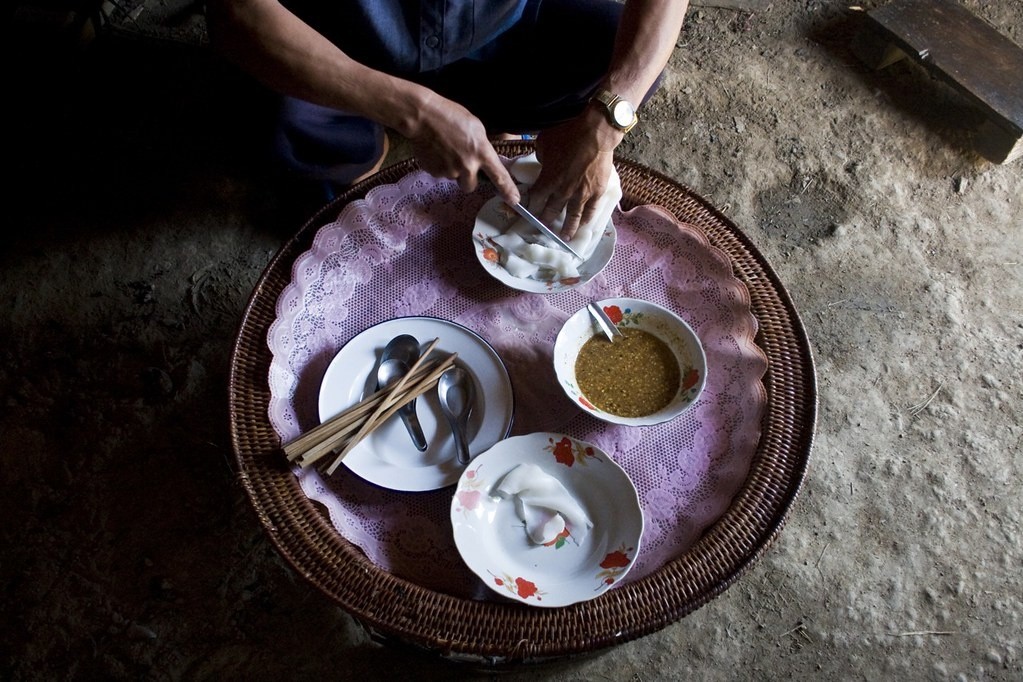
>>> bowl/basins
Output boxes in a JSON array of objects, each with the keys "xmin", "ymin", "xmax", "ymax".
[{"xmin": 553, "ymin": 296, "xmax": 707, "ymax": 425}]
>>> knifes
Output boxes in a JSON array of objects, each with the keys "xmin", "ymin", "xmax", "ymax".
[{"xmin": 478, "ymin": 170, "xmax": 580, "ymax": 260}]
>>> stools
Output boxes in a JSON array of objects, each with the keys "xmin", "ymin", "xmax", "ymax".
[{"xmin": 860, "ymin": 0, "xmax": 1023, "ymax": 168}]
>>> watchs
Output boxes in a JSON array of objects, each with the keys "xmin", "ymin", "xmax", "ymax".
[{"xmin": 589, "ymin": 88, "xmax": 638, "ymax": 133}]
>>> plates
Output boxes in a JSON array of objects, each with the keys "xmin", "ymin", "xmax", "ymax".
[
  {"xmin": 471, "ymin": 184, "xmax": 616, "ymax": 294},
  {"xmin": 449, "ymin": 432, "xmax": 644, "ymax": 608},
  {"xmin": 317, "ymin": 316, "xmax": 516, "ymax": 492}
]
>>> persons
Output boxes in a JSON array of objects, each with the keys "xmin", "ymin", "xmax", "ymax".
[{"xmin": 207, "ymin": 0, "xmax": 690, "ymax": 242}]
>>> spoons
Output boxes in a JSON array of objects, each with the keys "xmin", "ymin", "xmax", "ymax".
[
  {"xmin": 438, "ymin": 368, "xmax": 474, "ymax": 465},
  {"xmin": 374, "ymin": 333, "xmax": 421, "ymax": 394},
  {"xmin": 377, "ymin": 358, "xmax": 428, "ymax": 452}
]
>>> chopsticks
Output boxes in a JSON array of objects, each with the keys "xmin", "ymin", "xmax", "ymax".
[{"xmin": 279, "ymin": 336, "xmax": 458, "ymax": 477}]
